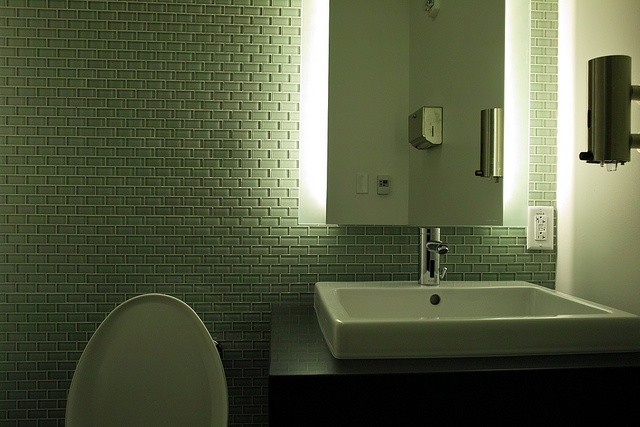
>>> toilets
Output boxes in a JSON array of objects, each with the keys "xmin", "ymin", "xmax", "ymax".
[{"xmin": 63, "ymin": 293, "xmax": 229, "ymax": 426}]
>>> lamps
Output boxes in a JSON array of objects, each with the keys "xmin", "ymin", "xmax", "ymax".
[
  {"xmin": 579, "ymin": 55, "xmax": 640, "ymax": 170},
  {"xmin": 475, "ymin": 108, "xmax": 503, "ymax": 182}
]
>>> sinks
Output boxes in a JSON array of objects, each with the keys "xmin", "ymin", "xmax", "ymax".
[{"xmin": 313, "ymin": 280, "xmax": 640, "ymax": 360}]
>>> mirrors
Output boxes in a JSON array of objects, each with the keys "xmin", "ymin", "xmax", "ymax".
[{"xmin": 298, "ymin": 0, "xmax": 532, "ymax": 226}]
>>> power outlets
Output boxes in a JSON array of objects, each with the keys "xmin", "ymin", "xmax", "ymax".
[{"xmin": 527, "ymin": 207, "xmax": 553, "ymax": 251}]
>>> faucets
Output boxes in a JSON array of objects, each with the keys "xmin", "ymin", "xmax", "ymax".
[{"xmin": 418, "ymin": 227, "xmax": 449, "ymax": 286}]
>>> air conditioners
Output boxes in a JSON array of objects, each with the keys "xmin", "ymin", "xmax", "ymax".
[{"xmin": 408, "ymin": 106, "xmax": 442, "ymax": 151}]
